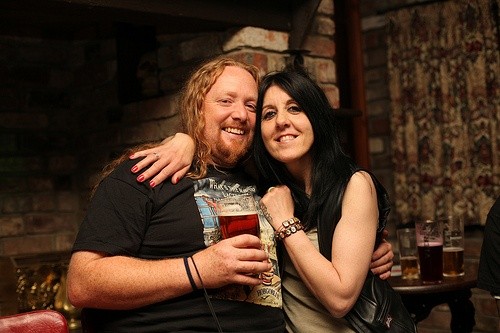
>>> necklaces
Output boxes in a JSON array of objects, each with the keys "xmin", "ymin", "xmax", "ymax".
[{"xmin": 306, "ymin": 192, "xmax": 312, "ymax": 198}]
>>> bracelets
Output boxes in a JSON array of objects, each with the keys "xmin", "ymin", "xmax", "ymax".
[
  {"xmin": 275, "ymin": 217, "xmax": 304, "ymax": 240},
  {"xmin": 184, "ymin": 255, "xmax": 222, "ymax": 333}
]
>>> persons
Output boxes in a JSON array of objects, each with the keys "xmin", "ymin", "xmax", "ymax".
[
  {"xmin": 129, "ymin": 65, "xmax": 418, "ymax": 333},
  {"xmin": 477, "ymin": 197, "xmax": 500, "ymax": 314},
  {"xmin": 66, "ymin": 58, "xmax": 395, "ymax": 333}
]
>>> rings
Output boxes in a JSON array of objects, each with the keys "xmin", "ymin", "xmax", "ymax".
[
  {"xmin": 267, "ymin": 187, "xmax": 275, "ymax": 192},
  {"xmin": 154, "ymin": 153, "xmax": 159, "ymax": 158}
]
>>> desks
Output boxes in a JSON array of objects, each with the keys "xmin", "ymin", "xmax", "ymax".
[{"xmin": 386, "ymin": 267, "xmax": 478, "ymax": 333}]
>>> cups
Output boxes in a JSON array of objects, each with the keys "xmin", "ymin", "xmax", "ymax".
[
  {"xmin": 398, "ymin": 229, "xmax": 419, "ymax": 287},
  {"xmin": 216, "ymin": 194, "xmax": 260, "ymax": 277},
  {"xmin": 415, "ymin": 219, "xmax": 445, "ymax": 285},
  {"xmin": 444, "ymin": 227, "xmax": 465, "ymax": 278},
  {"xmin": 16, "ymin": 265, "xmax": 81, "ymax": 331}
]
